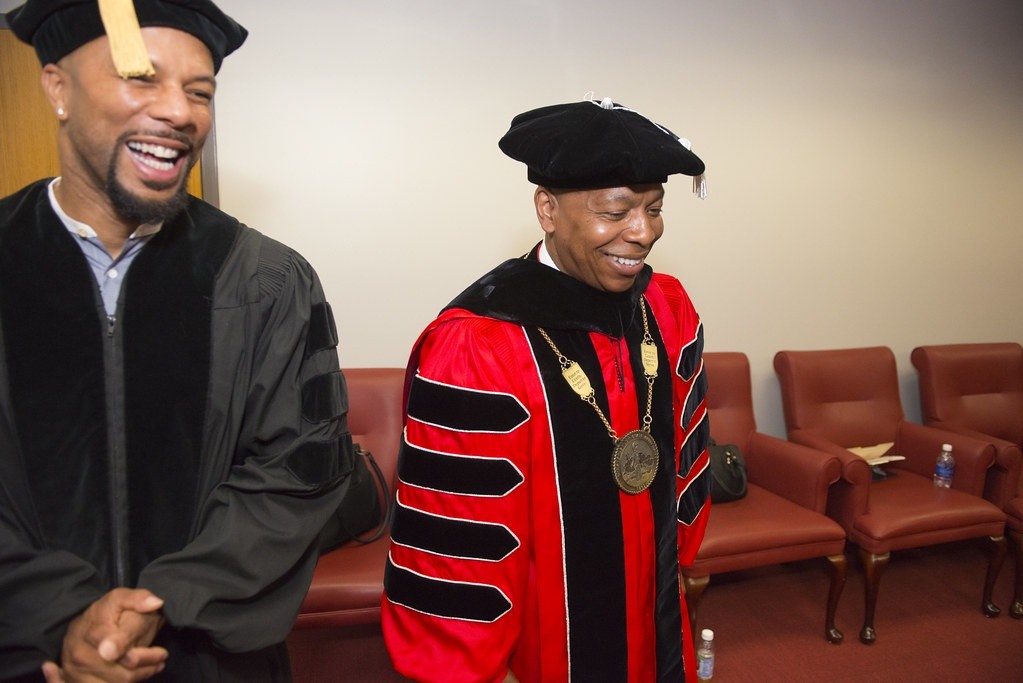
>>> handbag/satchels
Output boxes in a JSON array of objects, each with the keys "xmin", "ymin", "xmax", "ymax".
[
  {"xmin": 316, "ymin": 440, "xmax": 393, "ymax": 554},
  {"xmin": 704, "ymin": 438, "xmax": 747, "ymax": 501}
]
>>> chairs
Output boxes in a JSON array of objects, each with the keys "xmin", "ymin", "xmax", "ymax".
[
  {"xmin": 911, "ymin": 341, "xmax": 1023, "ymax": 619},
  {"xmin": 292, "ymin": 368, "xmax": 407, "ymax": 629},
  {"xmin": 775, "ymin": 344, "xmax": 1006, "ymax": 644},
  {"xmin": 683, "ymin": 352, "xmax": 848, "ymax": 643}
]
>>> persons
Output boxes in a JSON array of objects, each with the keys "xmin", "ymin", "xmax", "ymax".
[
  {"xmin": 0, "ymin": 0, "xmax": 360, "ymax": 683},
  {"xmin": 379, "ymin": 99, "xmax": 712, "ymax": 683}
]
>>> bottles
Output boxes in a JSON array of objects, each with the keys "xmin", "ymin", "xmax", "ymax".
[
  {"xmin": 933, "ymin": 443, "xmax": 955, "ymax": 489},
  {"xmin": 696, "ymin": 628, "xmax": 715, "ymax": 683}
]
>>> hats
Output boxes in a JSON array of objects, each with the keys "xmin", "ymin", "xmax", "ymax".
[
  {"xmin": 5, "ymin": 0, "xmax": 249, "ymax": 79},
  {"xmin": 498, "ymin": 96, "xmax": 708, "ymax": 201}
]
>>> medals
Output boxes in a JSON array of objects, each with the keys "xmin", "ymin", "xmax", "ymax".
[{"xmin": 613, "ymin": 428, "xmax": 660, "ymax": 494}]
